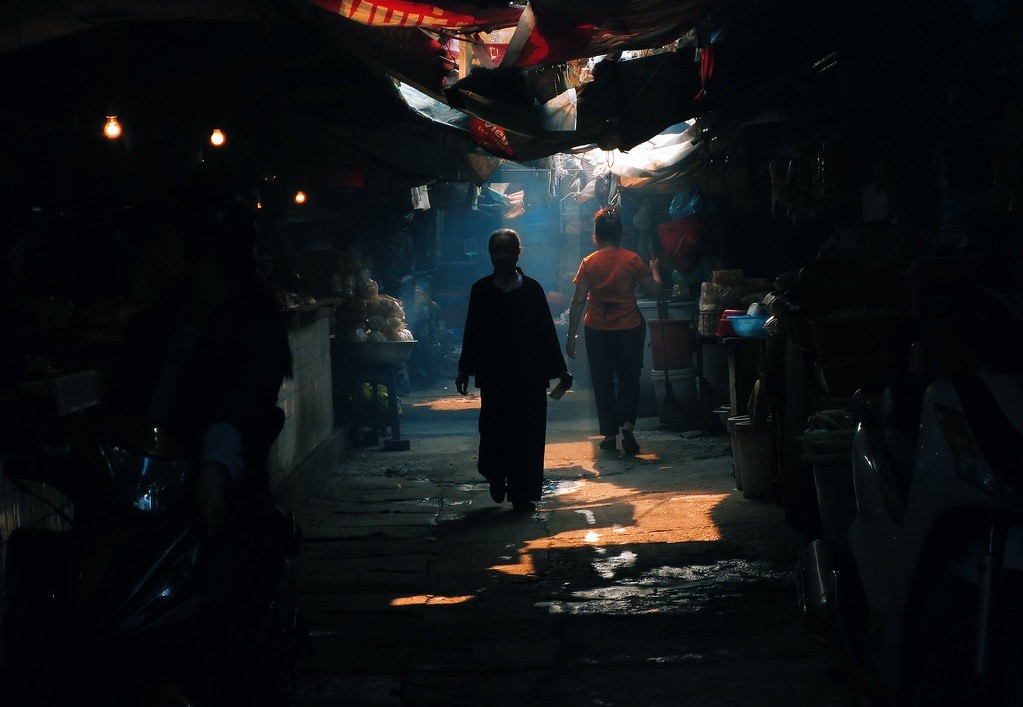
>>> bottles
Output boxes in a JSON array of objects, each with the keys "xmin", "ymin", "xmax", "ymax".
[{"xmin": 549, "ymin": 375, "xmax": 573, "ymax": 401}]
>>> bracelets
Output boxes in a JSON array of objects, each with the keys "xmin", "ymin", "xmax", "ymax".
[{"xmin": 567, "ymin": 333, "xmax": 578, "ymax": 339}]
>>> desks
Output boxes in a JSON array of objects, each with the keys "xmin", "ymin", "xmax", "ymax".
[{"xmin": 16, "ymin": 369, "xmax": 108, "ymax": 419}]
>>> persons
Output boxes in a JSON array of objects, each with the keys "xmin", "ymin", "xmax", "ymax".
[
  {"xmin": 456, "ymin": 228, "xmax": 573, "ymax": 513},
  {"xmin": 565, "ymin": 205, "xmax": 661, "ymax": 453}
]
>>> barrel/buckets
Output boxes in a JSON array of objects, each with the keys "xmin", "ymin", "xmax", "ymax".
[
  {"xmin": 647, "ymin": 318, "xmax": 692, "ymax": 370},
  {"xmin": 728, "ymin": 414, "xmax": 777, "ymax": 500},
  {"xmin": 651, "ymin": 370, "xmax": 700, "ymax": 430}
]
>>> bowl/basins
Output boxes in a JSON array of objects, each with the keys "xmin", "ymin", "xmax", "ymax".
[
  {"xmin": 332, "ymin": 340, "xmax": 419, "ymax": 367},
  {"xmin": 712, "ymin": 402, "xmax": 747, "ymax": 430},
  {"xmin": 726, "ymin": 314, "xmax": 770, "ymax": 337}
]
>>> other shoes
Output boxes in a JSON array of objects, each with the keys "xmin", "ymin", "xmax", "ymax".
[
  {"xmin": 598, "ymin": 438, "xmax": 639, "ymax": 454},
  {"xmin": 490, "ymin": 479, "xmax": 537, "ymax": 512}
]
